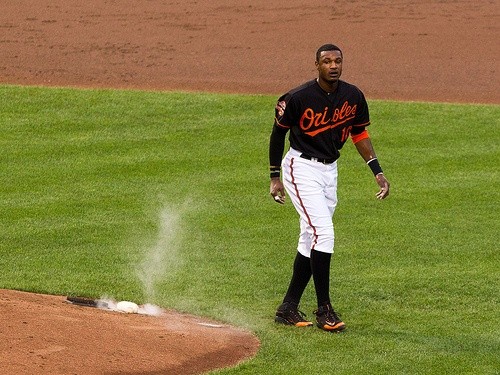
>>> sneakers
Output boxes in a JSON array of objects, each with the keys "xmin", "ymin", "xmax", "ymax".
[
  {"xmin": 313, "ymin": 302, "xmax": 346, "ymax": 333},
  {"xmin": 275, "ymin": 301, "xmax": 313, "ymax": 328}
]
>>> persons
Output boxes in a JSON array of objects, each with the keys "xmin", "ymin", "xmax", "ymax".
[{"xmin": 268, "ymin": 44, "xmax": 391, "ymax": 332}]
